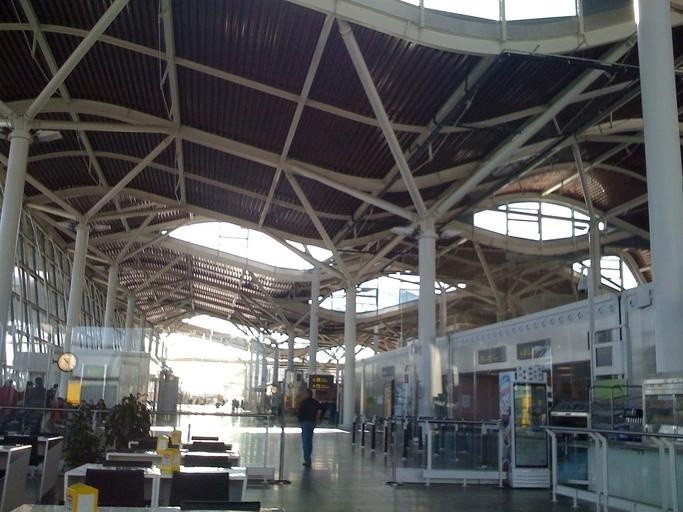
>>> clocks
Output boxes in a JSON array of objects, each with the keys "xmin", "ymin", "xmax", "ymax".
[{"xmin": 56, "ymin": 352, "xmax": 78, "ymax": 371}]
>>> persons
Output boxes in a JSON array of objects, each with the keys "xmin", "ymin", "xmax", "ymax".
[
  {"xmin": 86, "ymin": 399, "xmax": 100, "ymax": 410},
  {"xmin": 57, "ymin": 397, "xmax": 68, "ymax": 408},
  {"xmin": 29, "ymin": 377, "xmax": 46, "ymax": 415},
  {"xmin": 22, "ymin": 381, "xmax": 35, "ymax": 404},
  {"xmin": 296, "ymin": 387, "xmax": 327, "ymax": 468},
  {"xmin": 46, "ymin": 383, "xmax": 58, "ymax": 408},
  {"xmin": 96, "ymin": 398, "xmax": 107, "ymax": 409},
  {"xmin": 0, "ymin": 379, "xmax": 24, "ymax": 418},
  {"xmin": 78, "ymin": 399, "xmax": 91, "ymax": 409}
]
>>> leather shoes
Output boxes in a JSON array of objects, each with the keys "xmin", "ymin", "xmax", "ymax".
[{"xmin": 302, "ymin": 461, "xmax": 310, "ymax": 467}]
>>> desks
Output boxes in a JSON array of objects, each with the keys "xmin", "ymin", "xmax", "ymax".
[
  {"xmin": 63, "ymin": 440, "xmax": 248, "ymax": 507},
  {"xmin": 0, "ymin": 431, "xmax": 65, "ymax": 512}
]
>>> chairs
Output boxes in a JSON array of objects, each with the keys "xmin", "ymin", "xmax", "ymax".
[{"xmin": 85, "ymin": 437, "xmax": 230, "ymax": 507}]
